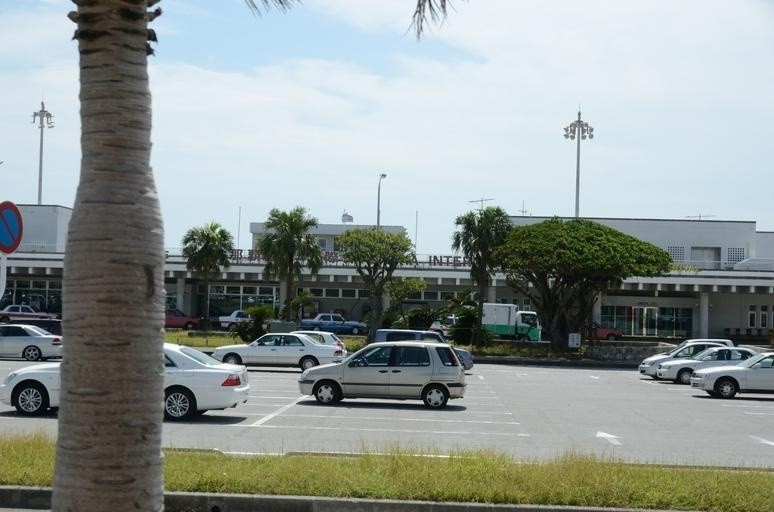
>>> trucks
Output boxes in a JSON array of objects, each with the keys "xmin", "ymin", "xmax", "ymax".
[{"xmin": 475, "ymin": 302, "xmax": 542, "ymax": 342}]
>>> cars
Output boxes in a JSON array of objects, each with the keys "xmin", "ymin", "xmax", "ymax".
[
  {"xmin": 637, "ymin": 338, "xmax": 774, "ymax": 399},
  {"xmin": 288, "ymin": 330, "xmax": 349, "ymax": 359},
  {"xmin": 211, "ymin": 333, "xmax": 345, "ymax": 372},
  {"xmin": 591, "ymin": 320, "xmax": 622, "ymax": 341},
  {"xmin": 300, "ymin": 313, "xmax": 368, "ymax": 336},
  {"xmin": 165, "ymin": 308, "xmax": 204, "ymax": 331},
  {"xmin": 218, "ymin": 310, "xmax": 268, "ymax": 330},
  {"xmin": 429, "ymin": 322, "xmax": 452, "ymax": 337},
  {"xmin": 298, "ymin": 341, "xmax": 466, "ymax": 410},
  {"xmin": 0, "ymin": 304, "xmax": 64, "ymax": 361},
  {"xmin": 23, "ymin": 293, "xmax": 46, "ymax": 304},
  {"xmin": 0, "ymin": 343, "xmax": 249, "ymax": 420}
]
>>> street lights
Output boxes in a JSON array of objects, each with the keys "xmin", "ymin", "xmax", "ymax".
[
  {"xmin": 564, "ymin": 109, "xmax": 595, "ymax": 218},
  {"xmin": 376, "ymin": 174, "xmax": 388, "ymax": 229},
  {"xmin": 32, "ymin": 98, "xmax": 55, "ymax": 206}
]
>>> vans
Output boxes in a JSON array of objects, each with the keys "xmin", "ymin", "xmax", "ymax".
[{"xmin": 375, "ymin": 328, "xmax": 473, "ymax": 372}]
